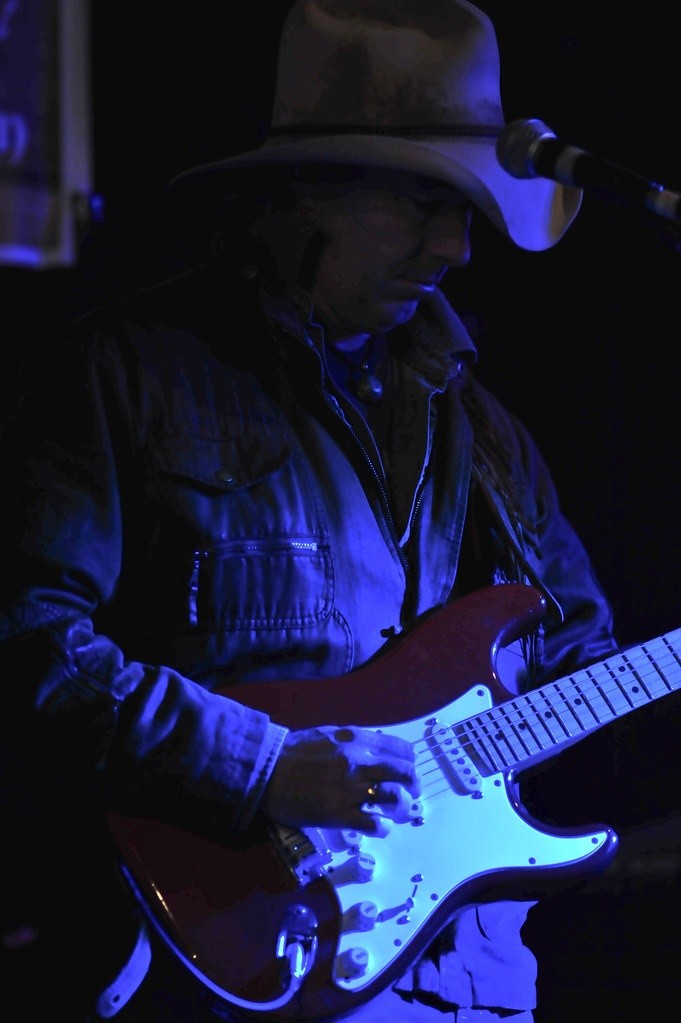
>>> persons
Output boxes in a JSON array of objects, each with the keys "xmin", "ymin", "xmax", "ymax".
[{"xmin": 0, "ymin": 0, "xmax": 627, "ymax": 1022}]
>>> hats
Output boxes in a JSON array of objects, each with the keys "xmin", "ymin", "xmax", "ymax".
[{"xmin": 169, "ymin": 0, "xmax": 587, "ymax": 252}]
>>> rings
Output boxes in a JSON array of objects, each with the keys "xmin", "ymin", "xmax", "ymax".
[{"xmin": 367, "ymin": 780, "xmax": 381, "ymax": 807}]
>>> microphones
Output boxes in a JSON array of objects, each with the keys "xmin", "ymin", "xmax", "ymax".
[{"xmin": 496, "ymin": 119, "xmax": 681, "ymax": 224}]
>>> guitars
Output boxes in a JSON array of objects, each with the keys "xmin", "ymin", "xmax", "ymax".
[{"xmin": 100, "ymin": 570, "xmax": 681, "ymax": 1022}]
